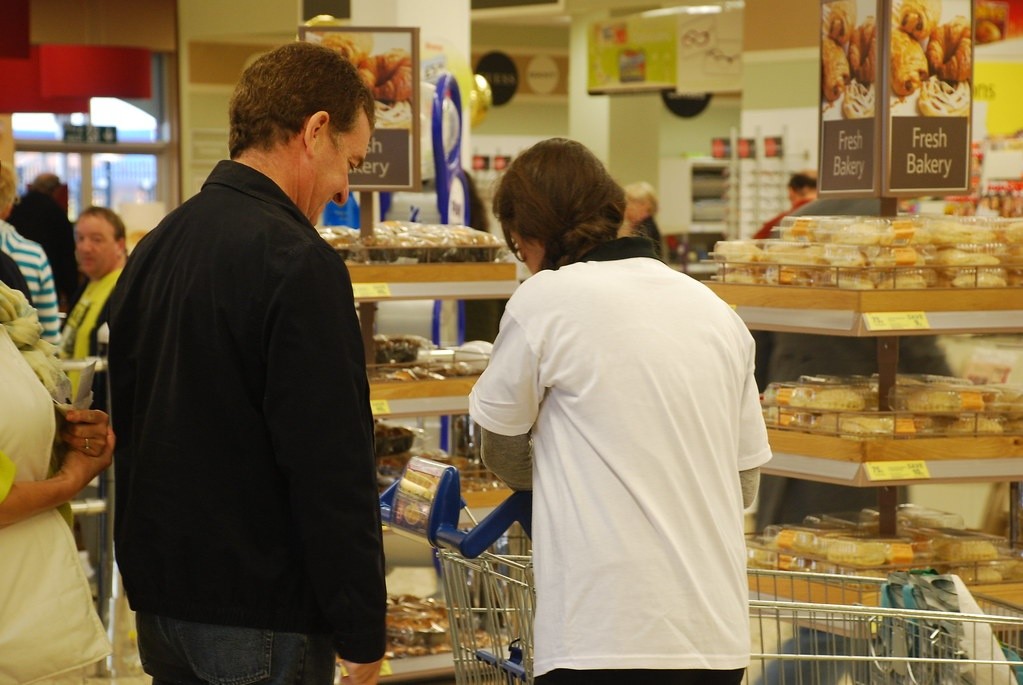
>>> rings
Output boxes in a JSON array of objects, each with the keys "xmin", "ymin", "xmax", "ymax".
[{"xmin": 85, "ymin": 438, "xmax": 89, "ymax": 449}]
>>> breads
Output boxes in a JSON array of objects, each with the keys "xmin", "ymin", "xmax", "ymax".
[
  {"xmin": 316, "ymin": 32, "xmax": 414, "ymax": 104},
  {"xmin": 758, "ymin": 364, "xmax": 1022, "ymax": 435},
  {"xmin": 745, "ymin": 511, "xmax": 1023, "ymax": 586},
  {"xmin": 710, "ymin": 215, "xmax": 1023, "ymax": 290},
  {"xmin": 822, "ymin": 0, "xmax": 973, "ymax": 119}
]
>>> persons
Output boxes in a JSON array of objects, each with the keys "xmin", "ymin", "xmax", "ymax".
[
  {"xmin": 0, "ymin": 280, "xmax": 116, "ymax": 685},
  {"xmin": 622, "ymin": 181, "xmax": 661, "ymax": 255},
  {"xmin": 110, "ymin": 41, "xmax": 388, "ymax": 685},
  {"xmin": 60, "ymin": 207, "xmax": 129, "ymax": 357},
  {"xmin": 753, "ymin": 172, "xmax": 817, "ymax": 238},
  {"xmin": 467, "ymin": 138, "xmax": 773, "ymax": 685},
  {"xmin": 6, "ymin": 174, "xmax": 80, "ymax": 300},
  {"xmin": 0, "ymin": 158, "xmax": 61, "ymax": 347}
]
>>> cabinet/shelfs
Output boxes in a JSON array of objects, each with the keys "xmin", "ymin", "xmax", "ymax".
[
  {"xmin": 704, "ymin": 255, "xmax": 1023, "ymax": 643},
  {"xmin": 336, "ymin": 262, "xmax": 521, "ymax": 685}
]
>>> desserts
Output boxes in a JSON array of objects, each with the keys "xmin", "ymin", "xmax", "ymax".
[
  {"xmin": 374, "ymin": 417, "xmax": 413, "ymax": 480},
  {"xmin": 380, "ymin": 590, "xmax": 506, "ymax": 658},
  {"xmin": 370, "ymin": 331, "xmax": 423, "ymax": 365},
  {"xmin": 316, "ymin": 220, "xmax": 507, "ymax": 265}
]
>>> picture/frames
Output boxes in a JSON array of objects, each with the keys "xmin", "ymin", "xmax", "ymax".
[
  {"xmin": 817, "ymin": 0, "xmax": 975, "ymax": 197},
  {"xmin": 298, "ymin": 26, "xmax": 422, "ymax": 191}
]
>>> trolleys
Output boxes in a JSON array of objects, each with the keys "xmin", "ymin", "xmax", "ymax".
[{"xmin": 378, "ymin": 457, "xmax": 1023, "ymax": 685}]
